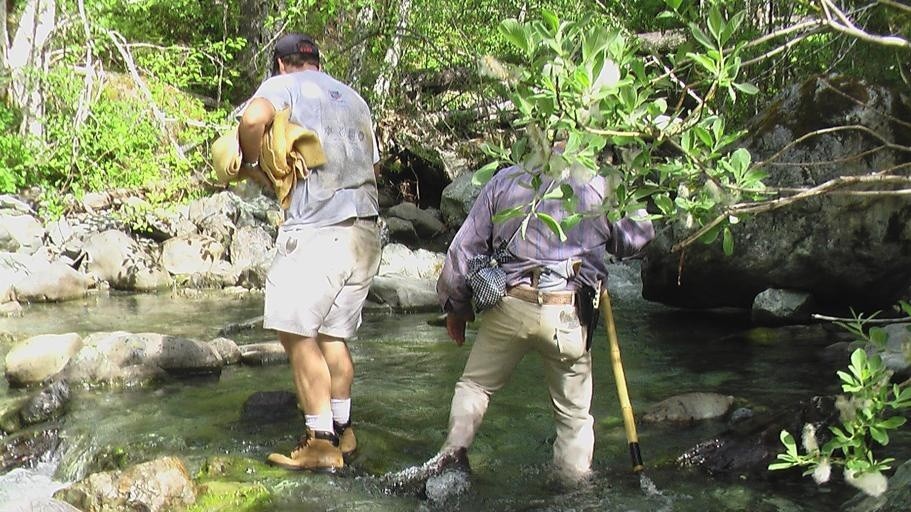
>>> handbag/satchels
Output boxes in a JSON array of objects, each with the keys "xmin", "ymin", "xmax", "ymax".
[{"xmin": 465, "ymin": 245, "xmax": 513, "ymax": 312}]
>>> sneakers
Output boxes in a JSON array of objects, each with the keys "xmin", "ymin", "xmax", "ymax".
[{"xmin": 267, "ymin": 414, "xmax": 356, "ymax": 475}]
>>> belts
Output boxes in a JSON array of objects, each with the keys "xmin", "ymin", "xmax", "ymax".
[{"xmin": 507, "ymin": 285, "xmax": 579, "ymax": 305}]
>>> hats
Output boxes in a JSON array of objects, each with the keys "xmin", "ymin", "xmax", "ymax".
[{"xmin": 272, "ymin": 33, "xmax": 320, "ymax": 75}]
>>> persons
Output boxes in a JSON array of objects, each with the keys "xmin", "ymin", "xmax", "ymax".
[
  {"xmin": 435, "ymin": 125, "xmax": 656, "ymax": 485},
  {"xmin": 237, "ymin": 32, "xmax": 386, "ymax": 471}
]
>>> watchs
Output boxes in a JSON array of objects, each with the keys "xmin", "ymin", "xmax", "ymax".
[{"xmin": 240, "ymin": 155, "xmax": 262, "ymax": 172}]
